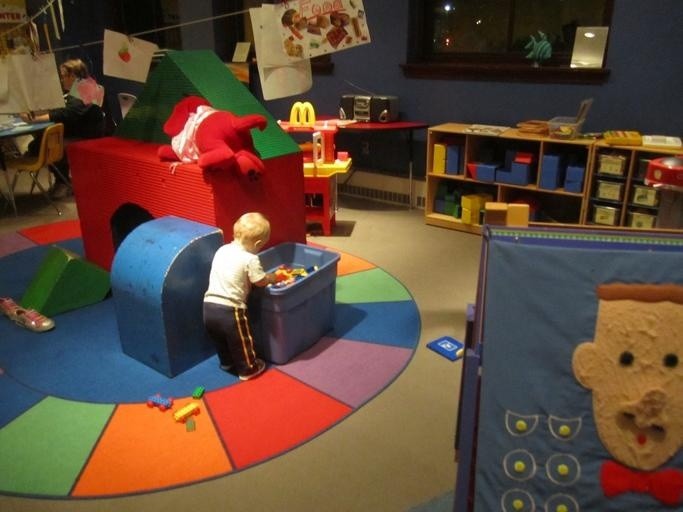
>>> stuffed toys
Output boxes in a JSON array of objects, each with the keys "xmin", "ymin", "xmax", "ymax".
[{"xmin": 156, "ymin": 95, "xmax": 272, "ymax": 188}]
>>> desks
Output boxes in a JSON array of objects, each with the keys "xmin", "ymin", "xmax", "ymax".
[
  {"xmin": 273, "ymin": 113, "xmax": 429, "ymax": 215},
  {"xmin": 0, "ymin": 118, "xmax": 58, "ymax": 199}
]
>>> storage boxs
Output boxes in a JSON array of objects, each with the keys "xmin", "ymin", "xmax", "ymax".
[{"xmin": 242, "ymin": 240, "xmax": 342, "ymax": 369}]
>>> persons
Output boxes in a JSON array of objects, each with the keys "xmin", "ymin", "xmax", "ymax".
[
  {"xmin": 201, "ymin": 212, "xmax": 278, "ymax": 382},
  {"xmin": 21, "ymin": 58, "xmax": 117, "ymax": 199}
]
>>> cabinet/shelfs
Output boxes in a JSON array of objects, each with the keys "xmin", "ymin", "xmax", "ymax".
[
  {"xmin": 422, "ymin": 121, "xmax": 597, "ymax": 242},
  {"xmin": 582, "ymin": 134, "xmax": 683, "ymax": 232}
]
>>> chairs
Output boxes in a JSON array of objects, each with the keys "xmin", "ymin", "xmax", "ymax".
[
  {"xmin": 0, "ymin": 141, "xmax": 23, "ymax": 221},
  {"xmin": 3, "ymin": 121, "xmax": 73, "ymax": 218},
  {"xmin": 116, "ymin": 90, "xmax": 136, "ymax": 120}
]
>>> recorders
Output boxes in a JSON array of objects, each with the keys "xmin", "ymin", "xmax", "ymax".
[{"xmin": 339, "ymin": 92, "xmax": 400, "ymax": 123}]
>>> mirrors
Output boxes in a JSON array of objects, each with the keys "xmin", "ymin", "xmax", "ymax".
[{"xmin": 568, "ymin": 25, "xmax": 610, "ymax": 71}]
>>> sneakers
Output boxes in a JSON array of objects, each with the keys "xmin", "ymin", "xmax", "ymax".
[
  {"xmin": 236, "ymin": 357, "xmax": 267, "ymax": 382},
  {"xmin": 218, "ymin": 363, "xmax": 233, "ymax": 371}
]
